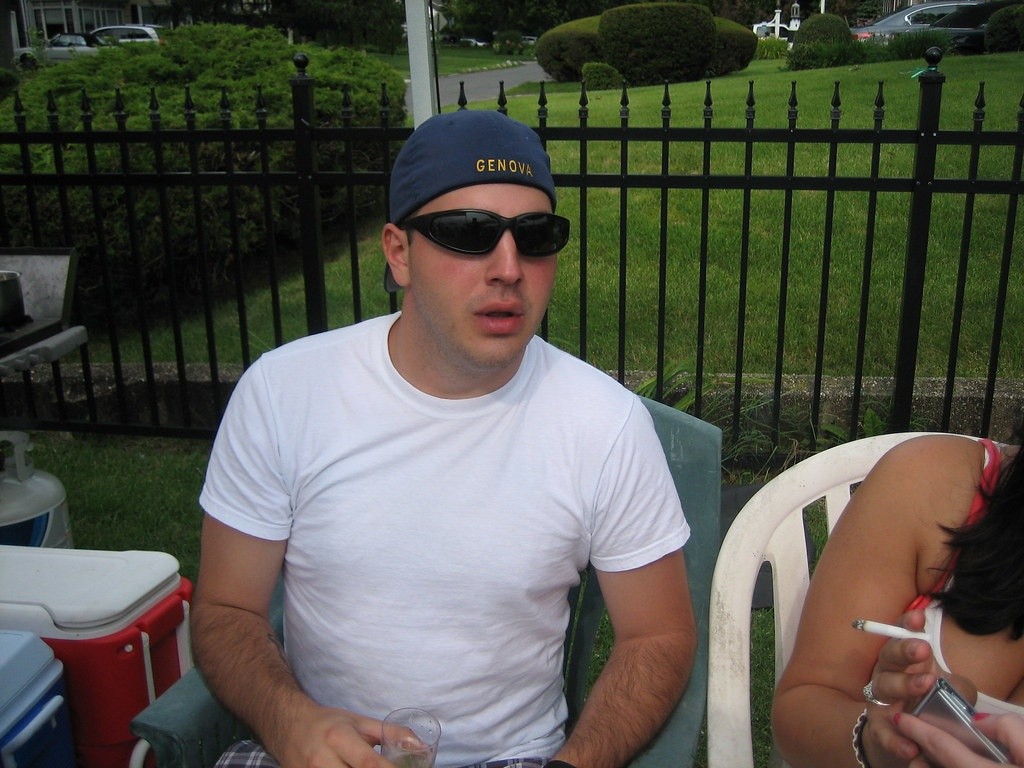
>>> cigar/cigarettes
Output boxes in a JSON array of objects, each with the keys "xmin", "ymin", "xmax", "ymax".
[{"xmin": 851, "ymin": 616, "xmax": 930, "ymax": 641}]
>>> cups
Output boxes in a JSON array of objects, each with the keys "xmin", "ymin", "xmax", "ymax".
[{"xmin": 380, "ymin": 707, "xmax": 442, "ymax": 768}]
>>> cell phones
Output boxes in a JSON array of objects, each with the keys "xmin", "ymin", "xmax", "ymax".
[{"xmin": 911, "ymin": 677, "xmax": 1014, "ymax": 764}]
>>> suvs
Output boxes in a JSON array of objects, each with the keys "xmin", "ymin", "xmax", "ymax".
[{"xmin": 89, "ymin": 23, "xmax": 165, "ymax": 46}]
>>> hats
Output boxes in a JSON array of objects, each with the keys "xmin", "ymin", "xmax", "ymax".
[{"xmin": 388, "ymin": 109, "xmax": 557, "ymax": 223}]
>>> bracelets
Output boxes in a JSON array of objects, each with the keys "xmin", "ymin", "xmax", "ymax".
[{"xmin": 852, "ymin": 707, "xmax": 869, "ymax": 768}]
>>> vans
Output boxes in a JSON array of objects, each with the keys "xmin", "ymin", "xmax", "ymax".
[{"xmin": 751, "ymin": 23, "xmax": 791, "ymax": 43}]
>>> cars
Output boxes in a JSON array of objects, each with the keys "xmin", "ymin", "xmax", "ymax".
[
  {"xmin": 850, "ymin": 0, "xmax": 989, "ymax": 56},
  {"xmin": 459, "ymin": 38, "xmax": 489, "ymax": 48},
  {"xmin": 12, "ymin": 32, "xmax": 118, "ymax": 72},
  {"xmin": 522, "ymin": 36, "xmax": 539, "ymax": 46}
]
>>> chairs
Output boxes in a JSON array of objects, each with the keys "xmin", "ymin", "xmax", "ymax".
[
  {"xmin": 128, "ymin": 395, "xmax": 720, "ymax": 768},
  {"xmin": 709, "ymin": 431, "xmax": 993, "ymax": 768}
]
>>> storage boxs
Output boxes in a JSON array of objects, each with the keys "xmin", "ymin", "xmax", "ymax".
[{"xmin": 0, "ymin": 542, "xmax": 194, "ymax": 768}]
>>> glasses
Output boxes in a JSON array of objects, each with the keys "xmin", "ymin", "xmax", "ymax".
[{"xmin": 398, "ymin": 208, "xmax": 571, "ymax": 257}]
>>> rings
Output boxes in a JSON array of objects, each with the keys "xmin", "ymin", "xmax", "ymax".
[{"xmin": 863, "ymin": 681, "xmax": 891, "ymax": 706}]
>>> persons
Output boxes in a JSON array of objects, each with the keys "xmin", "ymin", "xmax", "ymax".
[
  {"xmin": 771, "ymin": 396, "xmax": 1022, "ymax": 768},
  {"xmin": 191, "ymin": 114, "xmax": 697, "ymax": 768}
]
